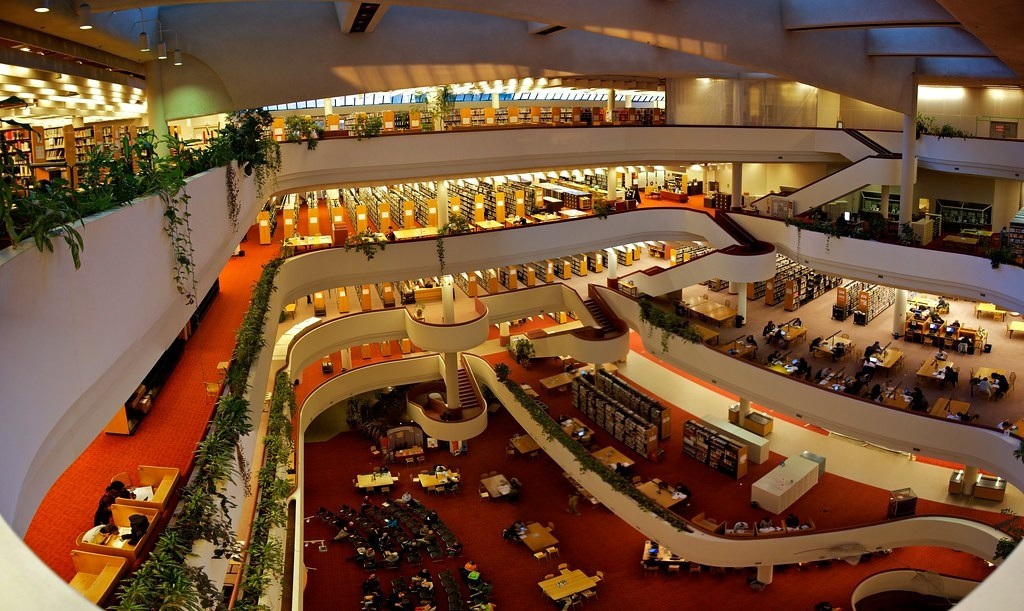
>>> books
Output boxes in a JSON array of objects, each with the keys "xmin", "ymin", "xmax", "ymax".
[
  {"xmin": 0, "ymin": 126, "xmax": 149, "ymax": 195},
  {"xmin": 314, "ymin": 244, "xmax": 637, "ymax": 318},
  {"xmin": 261, "ymin": 108, "xmax": 664, "ymax": 140},
  {"xmin": 683, "ymin": 422, "xmax": 738, "ymax": 478},
  {"xmin": 263, "ymin": 164, "xmax": 683, "ymax": 237},
  {"xmin": 571, "ymin": 372, "xmax": 663, "ymax": 460},
  {"xmin": 651, "ymin": 239, "xmax": 896, "ymax": 323}
]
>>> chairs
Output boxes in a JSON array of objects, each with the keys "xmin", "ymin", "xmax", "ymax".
[
  {"xmin": 111, "ymin": 472, "xmax": 133, "ymax": 488},
  {"xmin": 76, "ymin": 532, "xmax": 85, "ymax": 546},
  {"xmin": 317, "ymin": 446, "xmax": 680, "ymax": 611},
  {"xmin": 204, "ymin": 380, "xmax": 220, "ymax": 404}
]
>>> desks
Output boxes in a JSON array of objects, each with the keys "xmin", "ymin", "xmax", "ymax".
[
  {"xmin": 282, "ymin": 211, "xmax": 589, "ymax": 254},
  {"xmin": 511, "ymin": 418, "xmax": 686, "ymax": 600},
  {"xmin": 678, "ymin": 296, "xmax": 1024, "ymax": 440},
  {"xmin": 520, "ymin": 361, "xmax": 617, "ymax": 398},
  {"xmin": 396, "ymin": 447, "xmax": 423, "ymax": 458},
  {"xmin": 357, "ymin": 471, "xmax": 396, "ymax": 488},
  {"xmin": 943, "ymin": 229, "xmax": 993, "ymax": 251},
  {"xmin": 217, "ymin": 362, "xmax": 229, "ymax": 379},
  {"xmin": 481, "ymin": 474, "xmax": 513, "ymax": 498},
  {"xmin": 67, "ymin": 465, "xmax": 192, "ymax": 605},
  {"xmin": 418, "ymin": 470, "xmax": 458, "ymax": 487},
  {"xmin": 510, "ymin": 435, "xmax": 542, "ymax": 453}
]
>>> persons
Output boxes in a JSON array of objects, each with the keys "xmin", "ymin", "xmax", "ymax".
[
  {"xmin": 674, "ymin": 179, "xmax": 681, "ymax": 194},
  {"xmin": 759, "ymin": 516, "xmax": 773, "ymax": 528},
  {"xmin": 676, "ymin": 482, "xmax": 692, "ymax": 505},
  {"xmin": 365, "ymin": 226, "xmax": 375, "ymax": 236},
  {"xmin": 331, "ymin": 444, "xmax": 581, "ymax": 611},
  {"xmin": 82, "ymin": 524, "xmax": 120, "ymax": 546},
  {"xmin": 815, "ymin": 602, "xmax": 832, "ymax": 611},
  {"xmin": 385, "ymin": 226, "xmax": 395, "ymax": 237},
  {"xmin": 519, "ymin": 217, "xmax": 527, "ymax": 226},
  {"xmin": 96, "ymin": 480, "xmax": 136, "ymax": 525},
  {"xmin": 615, "ymin": 462, "xmax": 630, "ymax": 478},
  {"xmin": 734, "ymin": 521, "xmax": 749, "ymax": 530},
  {"xmin": 306, "ymin": 295, "xmax": 312, "ymax": 305},
  {"xmin": 745, "ymin": 297, "xmax": 1011, "ymax": 430},
  {"xmin": 627, "ymin": 185, "xmax": 636, "ymax": 199},
  {"xmin": 786, "ymin": 514, "xmax": 800, "ymax": 528},
  {"xmin": 292, "ymin": 229, "xmax": 300, "ymax": 237}
]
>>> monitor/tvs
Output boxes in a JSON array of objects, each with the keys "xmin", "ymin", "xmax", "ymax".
[
  {"xmin": 912, "ymin": 321, "xmax": 916, "ymax": 325},
  {"xmin": 930, "ymin": 324, "xmax": 938, "ymax": 330},
  {"xmin": 946, "ymin": 327, "xmax": 955, "ymax": 332}
]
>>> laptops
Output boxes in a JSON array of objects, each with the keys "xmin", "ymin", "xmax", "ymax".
[
  {"xmin": 791, "ymin": 358, "xmax": 799, "ymax": 366},
  {"xmin": 905, "ymin": 387, "xmax": 912, "ymax": 395}
]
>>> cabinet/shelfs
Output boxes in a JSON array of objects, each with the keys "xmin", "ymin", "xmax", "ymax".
[
  {"xmin": 572, "ymin": 369, "xmax": 749, "ymax": 479},
  {"xmin": 0, "ymin": 116, "xmax": 180, "ymax": 207},
  {"xmin": 747, "ymin": 251, "xmax": 894, "ymax": 324},
  {"xmin": 262, "ymin": 107, "xmax": 666, "ymax": 142},
  {"xmin": 258, "ymin": 180, "xmax": 544, "ymax": 244}
]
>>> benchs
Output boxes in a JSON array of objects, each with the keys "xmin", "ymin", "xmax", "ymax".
[{"xmin": 692, "ymin": 512, "xmax": 816, "ymax": 539}]
